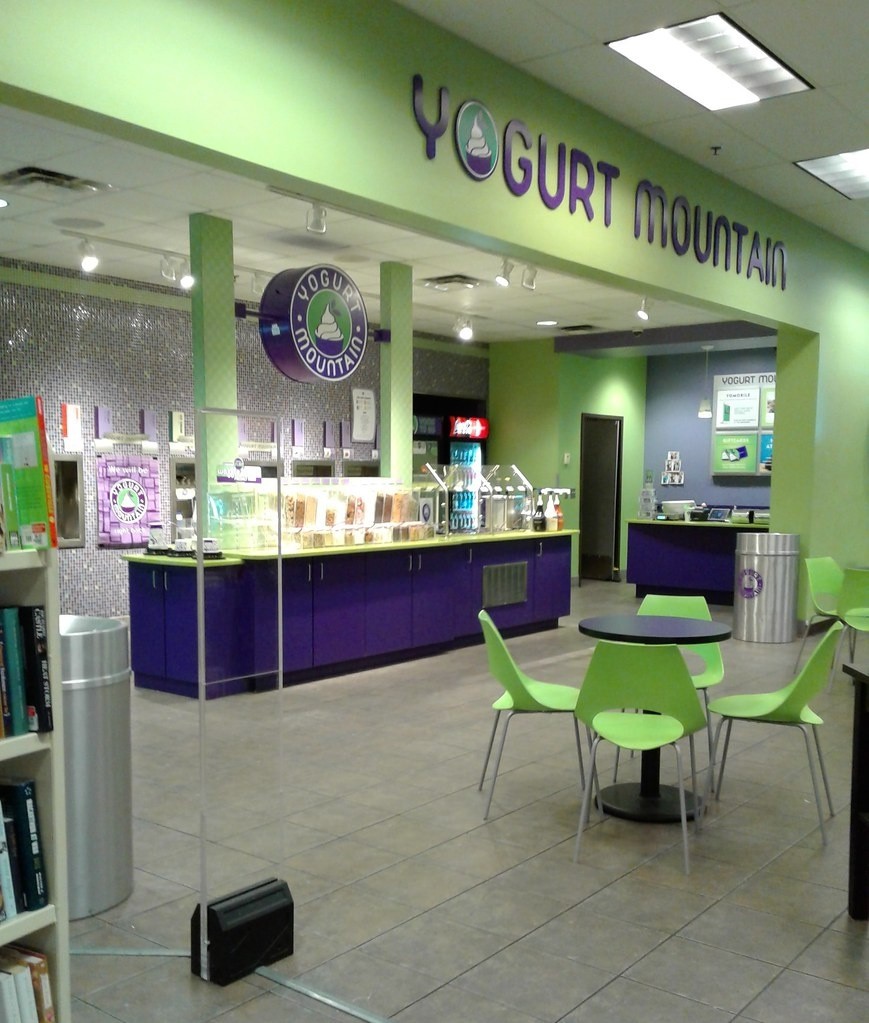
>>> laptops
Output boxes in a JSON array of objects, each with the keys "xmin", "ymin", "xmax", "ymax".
[{"xmin": 707, "ymin": 508, "xmax": 730, "ymax": 521}]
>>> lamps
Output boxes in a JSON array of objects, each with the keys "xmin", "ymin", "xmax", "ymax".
[
  {"xmin": 496, "ymin": 257, "xmax": 514, "ymax": 286},
  {"xmin": 180, "ymin": 257, "xmax": 193, "ymax": 288},
  {"xmin": 253, "ymin": 272, "xmax": 265, "ymax": 294},
  {"xmin": 637, "ymin": 297, "xmax": 650, "ymax": 320},
  {"xmin": 521, "ymin": 265, "xmax": 537, "ymax": 289},
  {"xmin": 452, "ymin": 314, "xmax": 473, "ymax": 340},
  {"xmin": 305, "ymin": 201, "xmax": 328, "ymax": 232},
  {"xmin": 77, "ymin": 238, "xmax": 98, "ymax": 270},
  {"xmin": 698, "ymin": 345, "xmax": 715, "ymax": 417}
]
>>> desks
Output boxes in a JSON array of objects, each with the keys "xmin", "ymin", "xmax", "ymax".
[
  {"xmin": 579, "ymin": 615, "xmax": 732, "ymax": 823},
  {"xmin": 624, "ymin": 519, "xmax": 769, "ymax": 606}
]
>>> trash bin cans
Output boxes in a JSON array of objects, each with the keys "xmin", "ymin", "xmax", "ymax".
[
  {"xmin": 58, "ymin": 612, "xmax": 134, "ymax": 923},
  {"xmin": 735, "ymin": 529, "xmax": 799, "ymax": 645}
]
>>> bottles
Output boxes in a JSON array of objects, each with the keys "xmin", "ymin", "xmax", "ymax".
[
  {"xmin": 176, "ymin": 511, "xmax": 184, "ymax": 528},
  {"xmin": 533, "ymin": 492, "xmax": 563, "ymax": 532},
  {"xmin": 149, "ymin": 525, "xmax": 164, "ymax": 549}
]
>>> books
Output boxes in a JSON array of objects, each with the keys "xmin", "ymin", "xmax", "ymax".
[
  {"xmin": 0, "ymin": 775, "xmax": 49, "ymax": 923},
  {"xmin": 0, "ymin": 942, "xmax": 56, "ymax": 1023},
  {"xmin": 0, "ymin": 605, "xmax": 54, "ymax": 738}
]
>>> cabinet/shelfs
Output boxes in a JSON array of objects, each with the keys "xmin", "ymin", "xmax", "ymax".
[
  {"xmin": 121, "ymin": 529, "xmax": 580, "ymax": 701},
  {"xmin": 0, "ymin": 420, "xmax": 72, "ymax": 1023}
]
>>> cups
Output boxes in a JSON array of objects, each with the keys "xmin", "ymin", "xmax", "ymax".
[
  {"xmin": 175, "ymin": 539, "xmax": 192, "ymax": 551},
  {"xmin": 203, "ymin": 539, "xmax": 219, "ymax": 553}
]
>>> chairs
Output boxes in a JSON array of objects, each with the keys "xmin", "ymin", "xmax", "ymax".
[
  {"xmin": 479, "ymin": 608, "xmax": 603, "ymax": 822},
  {"xmin": 793, "ymin": 556, "xmax": 869, "ymax": 693},
  {"xmin": 630, "ymin": 595, "xmax": 724, "ymax": 765},
  {"xmin": 707, "ymin": 621, "xmax": 843, "ymax": 845},
  {"xmin": 573, "ymin": 639, "xmax": 709, "ymax": 875}
]
